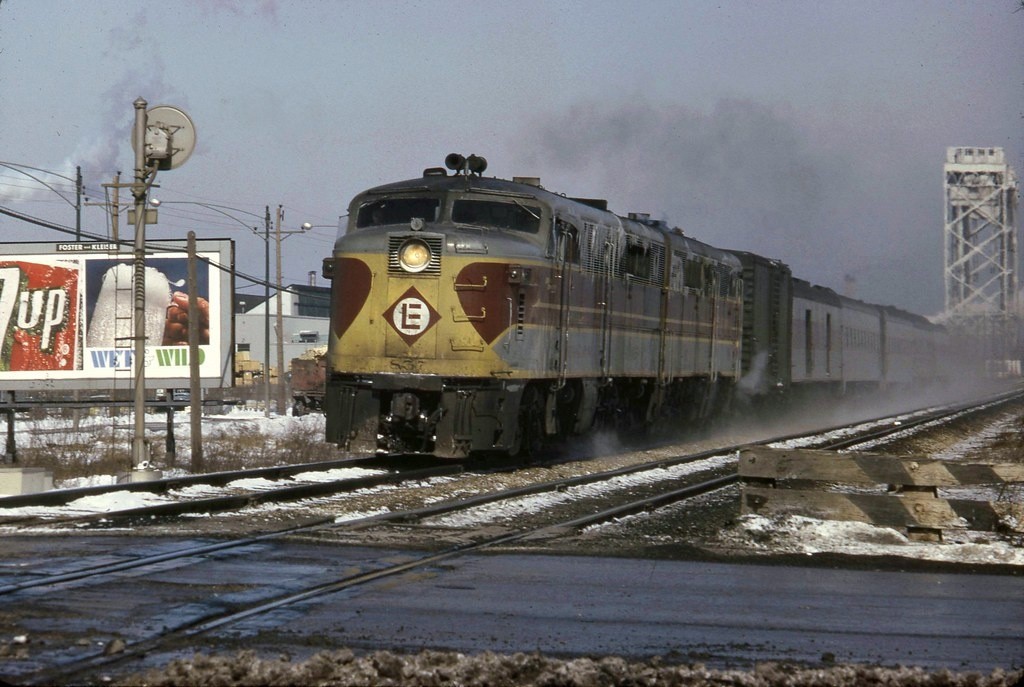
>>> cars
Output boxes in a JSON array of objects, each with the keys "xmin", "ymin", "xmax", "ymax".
[{"xmin": 154, "ymin": 388, "xmax": 191, "ymax": 413}]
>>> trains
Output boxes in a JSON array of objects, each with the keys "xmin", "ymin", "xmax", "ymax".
[{"xmin": 321, "ymin": 153, "xmax": 956, "ymax": 468}]
